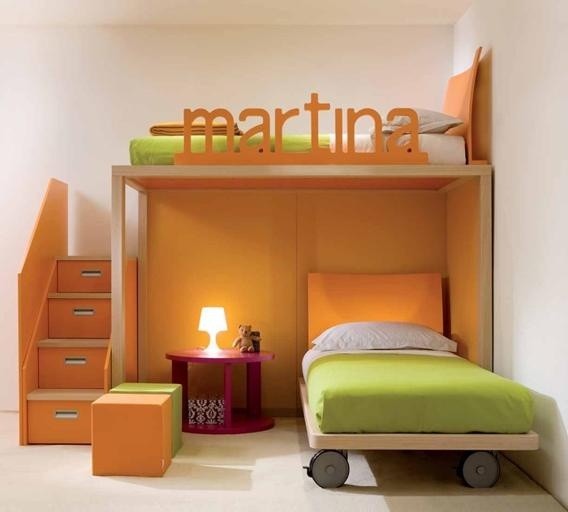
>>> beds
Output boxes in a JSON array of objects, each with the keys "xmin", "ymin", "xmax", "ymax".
[
  {"xmin": 298, "ymin": 266, "xmax": 540, "ymax": 491},
  {"xmin": 107, "ymin": 44, "xmax": 495, "ymax": 418}
]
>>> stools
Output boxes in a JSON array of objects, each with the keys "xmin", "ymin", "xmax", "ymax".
[
  {"xmin": 89, "ymin": 393, "xmax": 173, "ymax": 478},
  {"xmin": 108, "ymin": 379, "xmax": 184, "ymax": 459}
]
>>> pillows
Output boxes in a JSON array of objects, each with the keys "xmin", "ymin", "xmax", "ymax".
[
  {"xmin": 380, "ymin": 106, "xmax": 465, "ymax": 135},
  {"xmin": 312, "ymin": 318, "xmax": 459, "ymax": 354}
]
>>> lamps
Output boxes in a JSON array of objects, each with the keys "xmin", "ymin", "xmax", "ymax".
[{"xmin": 198, "ymin": 306, "xmax": 229, "ymax": 357}]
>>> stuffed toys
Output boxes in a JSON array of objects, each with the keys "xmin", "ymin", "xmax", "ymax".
[{"xmin": 234, "ymin": 324, "xmax": 261, "ymax": 353}]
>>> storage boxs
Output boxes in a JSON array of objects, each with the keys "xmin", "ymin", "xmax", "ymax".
[
  {"xmin": 25, "ymin": 399, "xmax": 90, "ymax": 447},
  {"xmin": 37, "ymin": 346, "xmax": 107, "ymax": 390},
  {"xmin": 48, "ymin": 299, "xmax": 111, "ymax": 340},
  {"xmin": 54, "ymin": 258, "xmax": 111, "ymax": 295}
]
